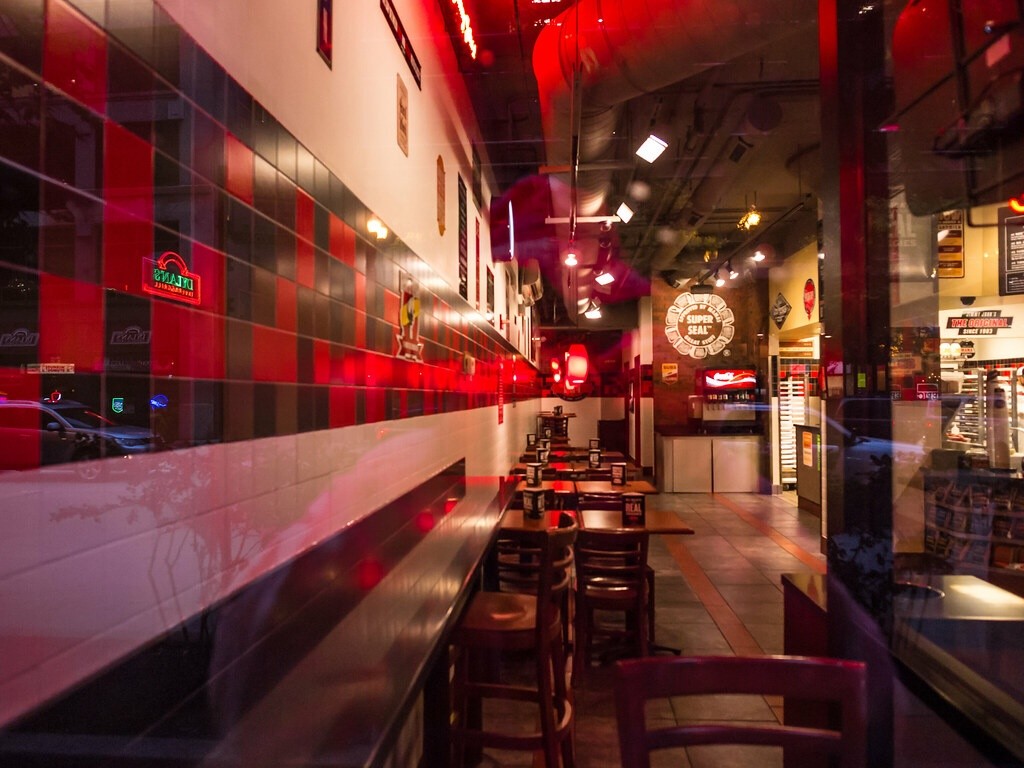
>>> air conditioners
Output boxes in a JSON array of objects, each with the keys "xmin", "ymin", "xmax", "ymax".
[{"xmin": 522, "ymin": 258, "xmax": 544, "ymax": 307}]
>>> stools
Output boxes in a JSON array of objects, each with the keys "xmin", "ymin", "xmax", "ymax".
[{"xmin": 420, "ymin": 510, "xmax": 580, "ymax": 768}]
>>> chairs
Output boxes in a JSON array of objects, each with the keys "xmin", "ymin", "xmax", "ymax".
[
  {"xmin": 489, "ymin": 410, "xmax": 651, "ymax": 661},
  {"xmin": 613, "ymin": 656, "xmax": 869, "ymax": 768}
]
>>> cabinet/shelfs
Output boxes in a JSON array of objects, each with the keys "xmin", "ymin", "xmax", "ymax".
[{"xmin": 657, "ymin": 432, "xmax": 768, "ymax": 495}]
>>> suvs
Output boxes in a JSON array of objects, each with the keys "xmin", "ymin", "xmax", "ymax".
[{"xmin": 1, "ymin": 396, "xmax": 161, "ymax": 473}]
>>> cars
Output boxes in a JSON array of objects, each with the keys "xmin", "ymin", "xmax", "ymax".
[{"xmin": 719, "ymin": 391, "xmax": 977, "ymax": 496}]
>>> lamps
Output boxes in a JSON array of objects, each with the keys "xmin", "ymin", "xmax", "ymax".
[{"xmin": 714, "ymin": 205, "xmax": 766, "ymax": 287}]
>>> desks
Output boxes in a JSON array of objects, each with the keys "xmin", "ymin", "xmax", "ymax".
[
  {"xmin": 509, "ymin": 410, "xmax": 658, "ymax": 494},
  {"xmin": 500, "ymin": 510, "xmax": 578, "ymax": 571},
  {"xmin": 580, "ymin": 508, "xmax": 695, "ymax": 656}
]
